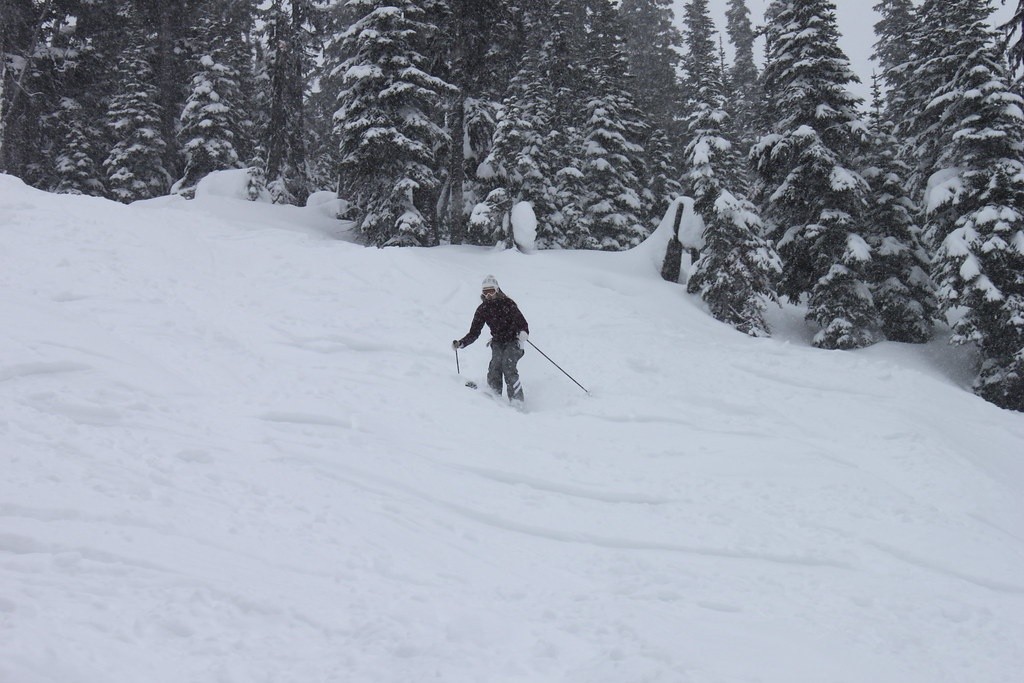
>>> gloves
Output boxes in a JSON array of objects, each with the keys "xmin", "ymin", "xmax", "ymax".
[
  {"xmin": 515, "ymin": 330, "xmax": 529, "ymax": 342},
  {"xmin": 452, "ymin": 340, "xmax": 464, "ymax": 351}
]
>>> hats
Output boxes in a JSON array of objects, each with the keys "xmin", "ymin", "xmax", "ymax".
[{"xmin": 481, "ymin": 274, "xmax": 499, "ymax": 293}]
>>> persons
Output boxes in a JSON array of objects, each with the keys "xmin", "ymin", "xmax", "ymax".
[{"xmin": 453, "ymin": 274, "xmax": 529, "ymax": 409}]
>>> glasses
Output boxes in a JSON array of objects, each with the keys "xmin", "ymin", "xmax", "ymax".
[{"xmin": 482, "ymin": 289, "xmax": 496, "ymax": 296}]
